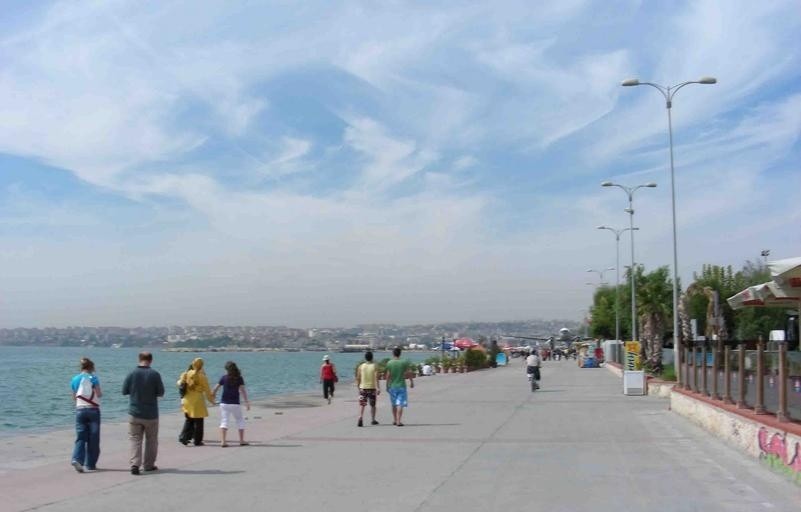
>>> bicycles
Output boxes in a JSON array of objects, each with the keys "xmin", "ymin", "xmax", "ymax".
[{"xmin": 527, "ymin": 366, "xmax": 541, "ymax": 393}]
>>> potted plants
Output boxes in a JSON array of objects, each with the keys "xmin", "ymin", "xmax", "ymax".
[
  {"xmin": 409, "ymin": 364, "xmax": 416, "ymax": 379},
  {"xmin": 440, "ymin": 355, "xmax": 447, "ymax": 373},
  {"xmin": 378, "ymin": 364, "xmax": 385, "ymax": 380},
  {"xmin": 429, "ymin": 359, "xmax": 436, "ymax": 374},
  {"xmin": 447, "ymin": 359, "xmax": 454, "ymax": 374},
  {"xmin": 462, "ymin": 350, "xmax": 488, "ymax": 374},
  {"xmin": 455, "ymin": 356, "xmax": 461, "ymax": 374}
]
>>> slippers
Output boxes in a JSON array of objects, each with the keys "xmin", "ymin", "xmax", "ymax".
[{"xmin": 395, "ymin": 421, "xmax": 403, "ymax": 425}]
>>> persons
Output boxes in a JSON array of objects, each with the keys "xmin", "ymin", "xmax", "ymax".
[
  {"xmin": 70, "ymin": 358, "xmax": 102, "ymax": 473},
  {"xmin": 121, "ymin": 351, "xmax": 165, "ymax": 475},
  {"xmin": 179, "ymin": 358, "xmax": 216, "ymax": 446},
  {"xmin": 385, "ymin": 348, "xmax": 414, "ymax": 426},
  {"xmin": 319, "ymin": 356, "xmax": 336, "ymax": 405},
  {"xmin": 527, "ymin": 349, "xmax": 541, "ymax": 389},
  {"xmin": 356, "ymin": 352, "xmax": 381, "ymax": 426},
  {"xmin": 211, "ymin": 361, "xmax": 251, "ymax": 447}
]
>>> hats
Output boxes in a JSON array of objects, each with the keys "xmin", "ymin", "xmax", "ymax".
[{"xmin": 321, "ymin": 354, "xmax": 333, "ymax": 363}]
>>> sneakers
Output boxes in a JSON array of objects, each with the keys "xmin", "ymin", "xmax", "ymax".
[
  {"xmin": 240, "ymin": 440, "xmax": 247, "ymax": 447},
  {"xmin": 357, "ymin": 417, "xmax": 364, "ymax": 427},
  {"xmin": 69, "ymin": 460, "xmax": 78, "ymax": 474},
  {"xmin": 145, "ymin": 465, "xmax": 156, "ymax": 471},
  {"xmin": 130, "ymin": 465, "xmax": 141, "ymax": 477},
  {"xmin": 370, "ymin": 419, "xmax": 378, "ymax": 425},
  {"xmin": 221, "ymin": 441, "xmax": 226, "ymax": 445}
]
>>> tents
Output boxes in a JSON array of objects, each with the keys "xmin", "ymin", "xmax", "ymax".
[{"xmin": 452, "ymin": 337, "xmax": 479, "ymax": 360}]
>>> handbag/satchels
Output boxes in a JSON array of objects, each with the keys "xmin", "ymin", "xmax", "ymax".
[
  {"xmin": 331, "ymin": 372, "xmax": 340, "ymax": 386},
  {"xmin": 178, "ymin": 379, "xmax": 190, "ymax": 397},
  {"xmin": 73, "ymin": 375, "xmax": 103, "ymax": 408}
]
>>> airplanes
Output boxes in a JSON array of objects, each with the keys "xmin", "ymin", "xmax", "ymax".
[{"xmin": 500, "ymin": 328, "xmax": 593, "ymax": 347}]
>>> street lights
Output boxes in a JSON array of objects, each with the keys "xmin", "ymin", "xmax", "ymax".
[
  {"xmin": 619, "ymin": 75, "xmax": 717, "ymax": 388},
  {"xmin": 600, "ymin": 181, "xmax": 658, "ymax": 343},
  {"xmin": 586, "ymin": 282, "xmax": 610, "ymax": 287},
  {"xmin": 585, "ymin": 266, "xmax": 616, "ymax": 287},
  {"xmin": 596, "ymin": 225, "xmax": 639, "ymax": 366}
]
